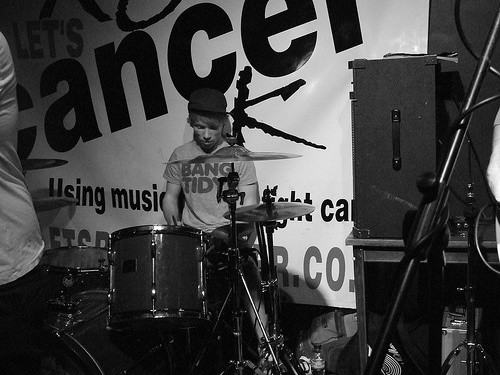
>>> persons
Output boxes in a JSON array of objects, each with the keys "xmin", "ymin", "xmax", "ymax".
[
  {"xmin": 0, "ymin": 31, "xmax": 55, "ymax": 375},
  {"xmin": 486, "ymin": 106, "xmax": 499, "ymax": 265},
  {"xmin": 162, "ymin": 88, "xmax": 278, "ymax": 375}
]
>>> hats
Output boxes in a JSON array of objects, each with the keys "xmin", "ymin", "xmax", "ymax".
[{"xmin": 188, "ymin": 88, "xmax": 231, "ymax": 114}]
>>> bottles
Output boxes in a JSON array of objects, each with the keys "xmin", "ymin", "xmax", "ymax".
[{"xmin": 311, "ymin": 343, "xmax": 325, "ymax": 375}]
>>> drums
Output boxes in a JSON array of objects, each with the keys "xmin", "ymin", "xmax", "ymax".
[
  {"xmin": 107, "ymin": 225, "xmax": 209, "ymax": 329},
  {"xmin": 34, "ymin": 246, "xmax": 110, "ymax": 297},
  {"xmin": 18, "ymin": 289, "xmax": 175, "ymax": 375}
]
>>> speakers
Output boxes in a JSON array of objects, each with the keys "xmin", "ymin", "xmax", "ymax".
[{"xmin": 351, "ymin": 56, "xmax": 465, "ymax": 239}]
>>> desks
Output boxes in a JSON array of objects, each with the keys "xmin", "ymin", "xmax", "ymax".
[{"xmin": 347, "ymin": 241, "xmax": 500, "ymax": 375}]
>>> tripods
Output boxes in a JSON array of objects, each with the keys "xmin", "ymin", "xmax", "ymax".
[
  {"xmin": 441, "ymin": 183, "xmax": 500, "ymax": 375},
  {"xmin": 189, "ymin": 163, "xmax": 300, "ymax": 375}
]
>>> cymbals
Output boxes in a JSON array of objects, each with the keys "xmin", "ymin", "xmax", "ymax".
[
  {"xmin": 33, "ymin": 197, "xmax": 78, "ymax": 212},
  {"xmin": 213, "ymin": 223, "xmax": 254, "ymax": 239},
  {"xmin": 162, "ymin": 145, "xmax": 303, "ymax": 163},
  {"xmin": 223, "ymin": 201, "xmax": 316, "ymax": 222},
  {"xmin": 20, "ymin": 158, "xmax": 68, "ymax": 171}
]
